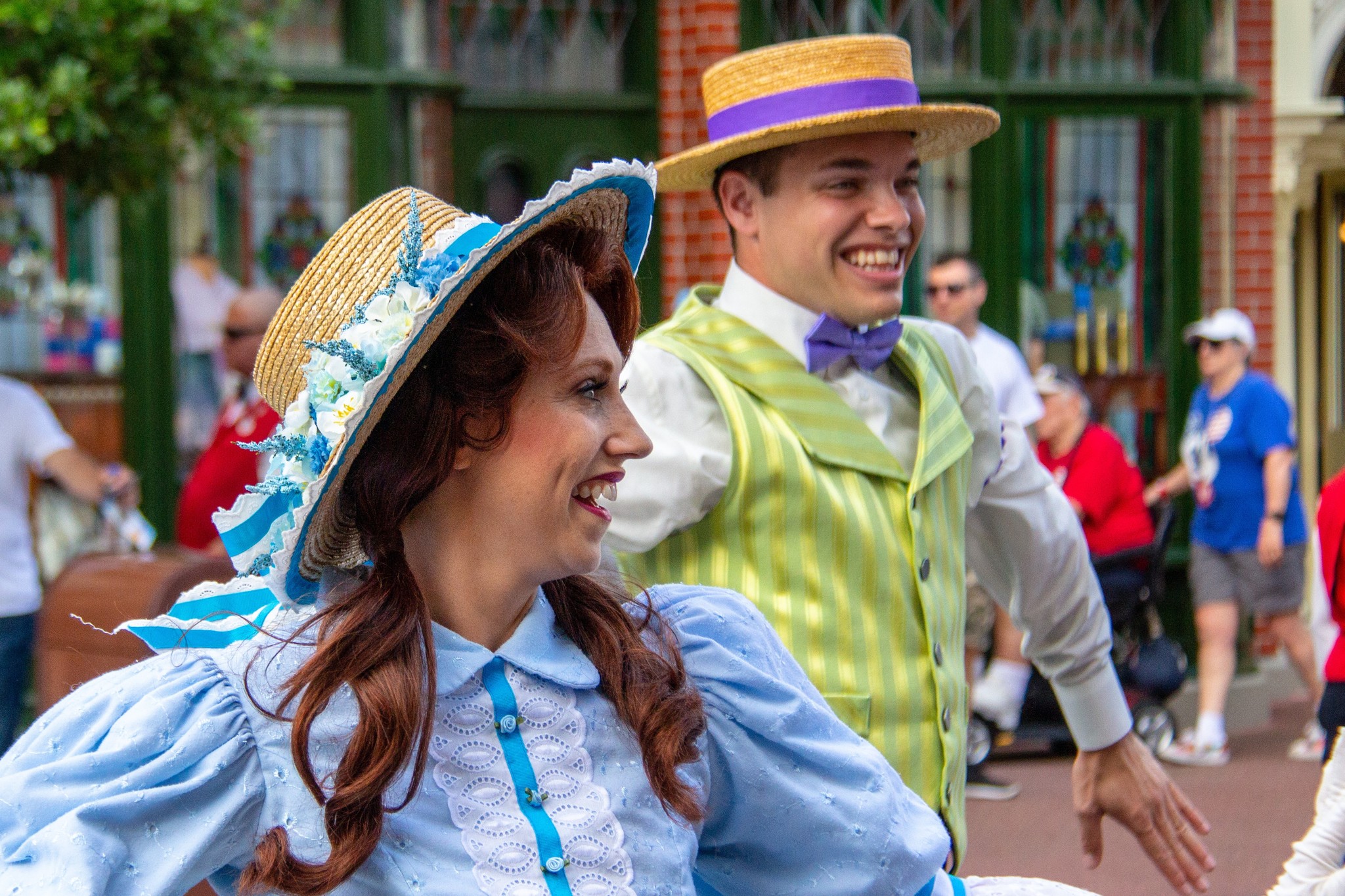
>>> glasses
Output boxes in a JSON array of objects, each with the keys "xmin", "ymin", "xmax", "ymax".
[
  {"xmin": 222, "ymin": 325, "xmax": 250, "ymax": 341},
  {"xmin": 1208, "ymin": 339, "xmax": 1220, "ymax": 348},
  {"xmin": 927, "ymin": 283, "xmax": 963, "ymax": 296}
]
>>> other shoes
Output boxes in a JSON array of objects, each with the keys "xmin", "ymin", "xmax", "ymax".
[
  {"xmin": 965, "ymin": 764, "xmax": 1018, "ymax": 800},
  {"xmin": 1288, "ymin": 730, "xmax": 1326, "ymax": 761},
  {"xmin": 1163, "ymin": 736, "xmax": 1229, "ymax": 767},
  {"xmin": 967, "ymin": 679, "xmax": 1020, "ymax": 729}
]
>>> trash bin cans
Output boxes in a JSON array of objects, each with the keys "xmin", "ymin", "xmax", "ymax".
[{"xmin": 34, "ymin": 545, "xmax": 234, "ymax": 716}]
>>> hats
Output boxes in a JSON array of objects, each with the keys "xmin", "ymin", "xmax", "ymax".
[
  {"xmin": 650, "ymin": 32, "xmax": 1000, "ymax": 194},
  {"xmin": 1185, "ymin": 307, "xmax": 1257, "ymax": 350},
  {"xmin": 1035, "ymin": 361, "xmax": 1095, "ymax": 411},
  {"xmin": 210, "ymin": 156, "xmax": 663, "ymax": 610}
]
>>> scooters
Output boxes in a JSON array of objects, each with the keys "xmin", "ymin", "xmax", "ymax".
[{"xmin": 959, "ymin": 489, "xmax": 1188, "ymax": 777}]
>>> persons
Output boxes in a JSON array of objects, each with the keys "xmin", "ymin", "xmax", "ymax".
[
  {"xmin": 171, "ymin": 228, "xmax": 239, "ymax": 452},
  {"xmin": 179, "ymin": 288, "xmax": 284, "ymax": 559},
  {"xmin": 0, "ymin": 157, "xmax": 1100, "ymax": 896},
  {"xmin": 586, "ymin": 34, "xmax": 1214, "ymax": 896},
  {"xmin": 922, "ymin": 250, "xmax": 1345, "ymax": 896},
  {"xmin": 0, "ymin": 377, "xmax": 139, "ymax": 760}
]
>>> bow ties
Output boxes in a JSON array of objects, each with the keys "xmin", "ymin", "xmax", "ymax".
[{"xmin": 803, "ymin": 313, "xmax": 902, "ymax": 383}]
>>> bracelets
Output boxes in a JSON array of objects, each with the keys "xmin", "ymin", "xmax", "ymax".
[{"xmin": 1264, "ymin": 513, "xmax": 1284, "ymax": 520}]
